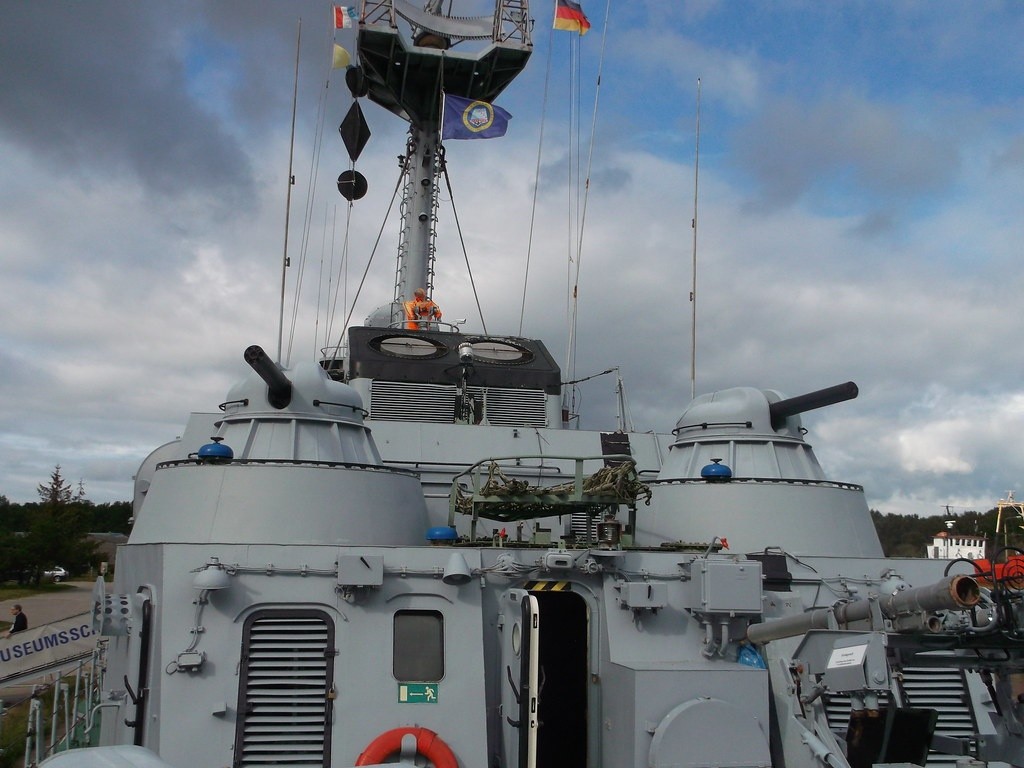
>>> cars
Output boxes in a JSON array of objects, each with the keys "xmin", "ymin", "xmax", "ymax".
[{"xmin": 45, "ymin": 566, "xmax": 69, "ymax": 582}]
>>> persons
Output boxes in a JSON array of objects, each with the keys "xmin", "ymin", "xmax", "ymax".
[
  {"xmin": 5, "ymin": 605, "xmax": 27, "ymax": 638},
  {"xmin": 405, "ymin": 288, "xmax": 442, "ymax": 330}
]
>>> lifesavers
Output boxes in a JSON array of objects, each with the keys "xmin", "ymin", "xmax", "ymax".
[{"xmin": 355, "ymin": 727, "xmax": 459, "ymax": 768}]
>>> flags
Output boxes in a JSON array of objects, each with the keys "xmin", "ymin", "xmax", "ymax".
[
  {"xmin": 555, "ymin": 0, "xmax": 590, "ymax": 35},
  {"xmin": 332, "ymin": 44, "xmax": 349, "ymax": 70},
  {"xmin": 335, "ymin": 6, "xmax": 357, "ymax": 30},
  {"xmin": 443, "ymin": 93, "xmax": 512, "ymax": 143}
]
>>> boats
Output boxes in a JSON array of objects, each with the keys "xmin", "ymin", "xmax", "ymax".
[{"xmin": 24, "ymin": 0, "xmax": 1023, "ymax": 768}]
